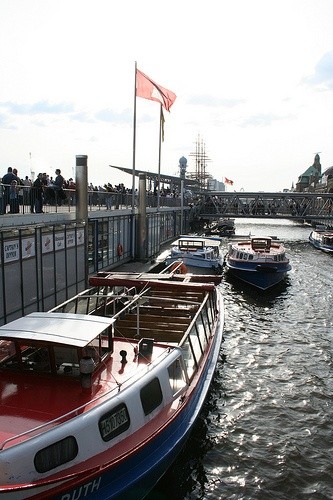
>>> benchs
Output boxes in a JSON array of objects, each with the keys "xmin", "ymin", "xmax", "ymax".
[{"xmin": 114, "ymin": 306, "xmax": 191, "ymax": 380}]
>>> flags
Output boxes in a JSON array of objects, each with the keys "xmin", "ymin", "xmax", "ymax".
[
  {"xmin": 225, "ymin": 176, "xmax": 233, "ymax": 186},
  {"xmin": 135, "ymin": 68, "xmax": 177, "ymax": 112}
]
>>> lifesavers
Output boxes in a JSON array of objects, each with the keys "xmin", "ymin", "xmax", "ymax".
[{"xmin": 117, "ymin": 244, "xmax": 124, "ymax": 256}]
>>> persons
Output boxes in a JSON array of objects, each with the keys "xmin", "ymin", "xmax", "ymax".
[
  {"xmin": 88, "ymin": 182, "xmax": 140, "ymax": 210},
  {"xmin": 144, "ymin": 187, "xmax": 196, "ymax": 207},
  {"xmin": 203, "ymin": 202, "xmax": 332, "ymax": 216},
  {"xmin": 0, "ymin": 167, "xmax": 25, "ymax": 215},
  {"xmin": 19, "ymin": 169, "xmax": 76, "ymax": 213}
]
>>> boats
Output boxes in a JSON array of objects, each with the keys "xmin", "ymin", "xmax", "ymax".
[
  {"xmin": 0, "ymin": 260, "xmax": 225, "ymax": 500},
  {"xmin": 309, "ymin": 228, "xmax": 333, "ymax": 255},
  {"xmin": 163, "ymin": 233, "xmax": 228, "ymax": 275},
  {"xmin": 224, "ymin": 235, "xmax": 292, "ymax": 291}
]
